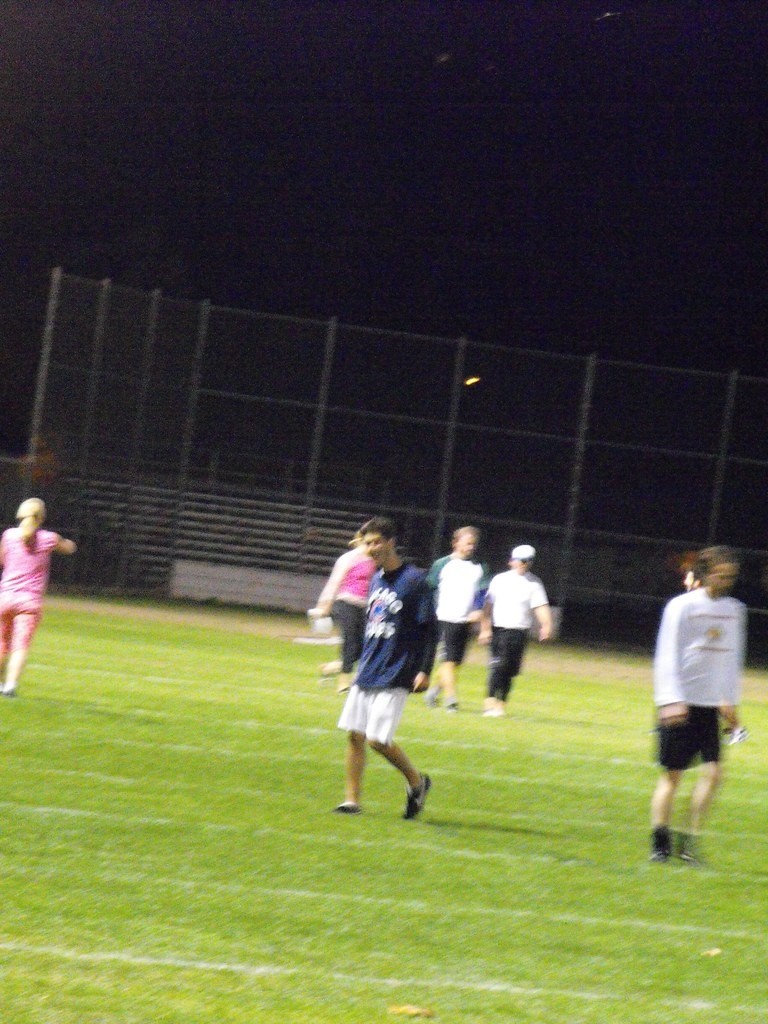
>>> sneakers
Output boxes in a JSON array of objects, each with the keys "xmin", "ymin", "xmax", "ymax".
[
  {"xmin": 333, "ymin": 802, "xmax": 361, "ymax": 814},
  {"xmin": 403, "ymin": 775, "xmax": 431, "ymax": 819}
]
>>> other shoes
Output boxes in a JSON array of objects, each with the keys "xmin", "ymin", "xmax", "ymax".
[
  {"xmin": 3, "ymin": 682, "xmax": 16, "ymax": 695},
  {"xmin": 650, "ymin": 850, "xmax": 670, "ymax": 862},
  {"xmin": 679, "ymin": 850, "xmax": 695, "ymax": 864}
]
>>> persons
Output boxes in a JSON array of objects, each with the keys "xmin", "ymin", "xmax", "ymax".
[
  {"xmin": 308, "ymin": 529, "xmax": 378, "ymax": 692},
  {"xmin": 648, "ymin": 547, "xmax": 747, "ymax": 862},
  {"xmin": 328, "ymin": 517, "xmax": 438, "ymax": 819},
  {"xmin": 0, "ymin": 498, "xmax": 76, "ymax": 695},
  {"xmin": 423, "ymin": 527, "xmax": 490, "ymax": 708},
  {"xmin": 477, "ymin": 543, "xmax": 554, "ymax": 719}
]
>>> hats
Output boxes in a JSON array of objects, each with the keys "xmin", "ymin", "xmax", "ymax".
[{"xmin": 512, "ymin": 545, "xmax": 536, "ymax": 560}]
